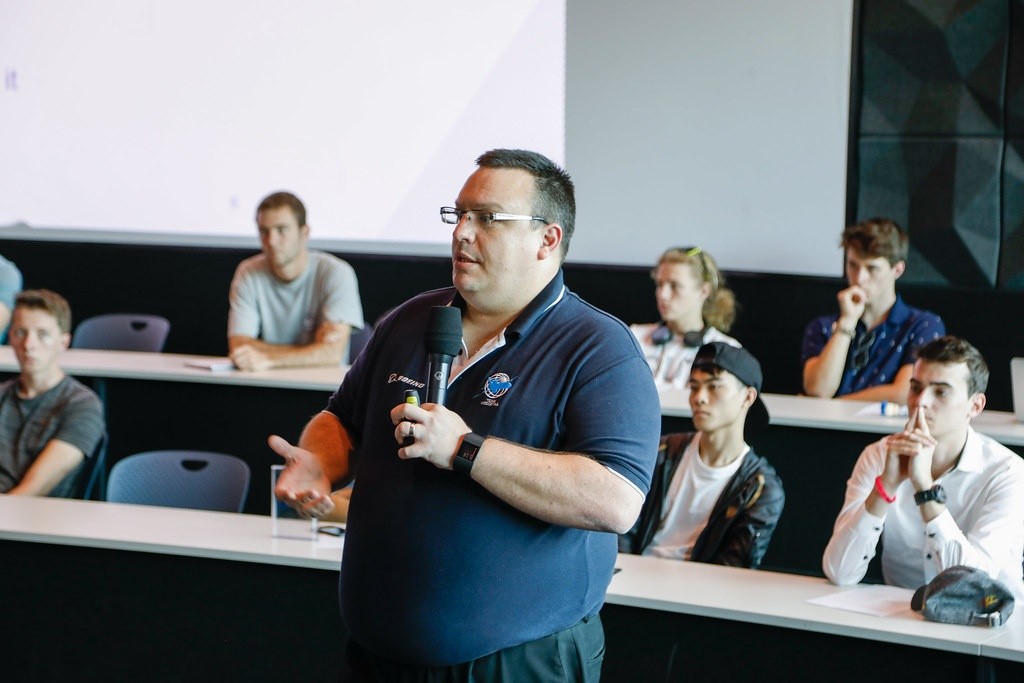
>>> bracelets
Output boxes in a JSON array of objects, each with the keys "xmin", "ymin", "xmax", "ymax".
[
  {"xmin": 453, "ymin": 432, "xmax": 488, "ymax": 477},
  {"xmin": 875, "ymin": 476, "xmax": 896, "ymax": 503}
]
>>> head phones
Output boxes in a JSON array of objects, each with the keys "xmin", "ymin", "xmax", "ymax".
[{"xmin": 651, "ymin": 316, "xmax": 710, "ymax": 345}]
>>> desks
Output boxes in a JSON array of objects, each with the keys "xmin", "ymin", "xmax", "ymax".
[
  {"xmin": 0, "ymin": 337, "xmax": 352, "ymax": 515},
  {"xmin": 0, "ymin": 491, "xmax": 348, "ymax": 683},
  {"xmin": 657, "ymin": 381, "xmax": 1024, "ymax": 582},
  {"xmin": 604, "ymin": 552, "xmax": 1024, "ymax": 683}
]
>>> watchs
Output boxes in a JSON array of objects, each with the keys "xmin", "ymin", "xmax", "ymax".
[
  {"xmin": 914, "ymin": 484, "xmax": 946, "ymax": 505},
  {"xmin": 830, "ymin": 321, "xmax": 856, "ymax": 341}
]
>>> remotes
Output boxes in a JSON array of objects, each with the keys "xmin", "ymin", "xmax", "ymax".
[{"xmin": 404, "ymin": 389, "xmax": 421, "ymax": 446}]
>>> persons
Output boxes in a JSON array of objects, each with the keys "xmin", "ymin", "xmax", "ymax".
[
  {"xmin": 797, "ymin": 219, "xmax": 945, "ymax": 405},
  {"xmin": 228, "ymin": 192, "xmax": 365, "ymax": 371},
  {"xmin": 822, "ymin": 339, "xmax": 1024, "ymax": 592},
  {"xmin": 0, "ymin": 290, "xmax": 105, "ymax": 496},
  {"xmin": 628, "ymin": 247, "xmax": 742, "ymax": 413},
  {"xmin": 266, "ymin": 146, "xmax": 664, "ymax": 683},
  {"xmin": 616, "ymin": 344, "xmax": 785, "ymax": 569},
  {"xmin": 0, "ymin": 254, "xmax": 24, "ymax": 345}
]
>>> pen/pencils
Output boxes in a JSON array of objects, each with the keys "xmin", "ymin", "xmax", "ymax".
[{"xmin": 186, "ymin": 363, "xmax": 213, "ymax": 372}]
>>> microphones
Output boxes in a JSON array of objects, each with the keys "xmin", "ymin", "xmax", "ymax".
[{"xmin": 422, "ymin": 306, "xmax": 463, "ymax": 408}]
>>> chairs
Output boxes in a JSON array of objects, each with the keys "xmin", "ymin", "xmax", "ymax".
[
  {"xmin": 71, "ymin": 314, "xmax": 172, "ymax": 411},
  {"xmin": 105, "ymin": 450, "xmax": 250, "ymax": 514},
  {"xmin": 72, "ymin": 424, "xmax": 108, "ymax": 499},
  {"xmin": 270, "ymin": 464, "xmax": 355, "ymax": 521}
]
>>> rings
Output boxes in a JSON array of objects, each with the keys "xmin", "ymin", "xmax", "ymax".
[{"xmin": 410, "ymin": 422, "xmax": 415, "ymax": 435}]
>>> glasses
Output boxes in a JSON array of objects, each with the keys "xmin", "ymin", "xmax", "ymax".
[
  {"xmin": 679, "ymin": 246, "xmax": 710, "ymax": 283},
  {"xmin": 440, "ymin": 206, "xmax": 549, "ymax": 227}
]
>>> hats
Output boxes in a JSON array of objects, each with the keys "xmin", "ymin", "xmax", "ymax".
[
  {"xmin": 691, "ymin": 342, "xmax": 770, "ymax": 438},
  {"xmin": 911, "ymin": 564, "xmax": 1015, "ymax": 628}
]
